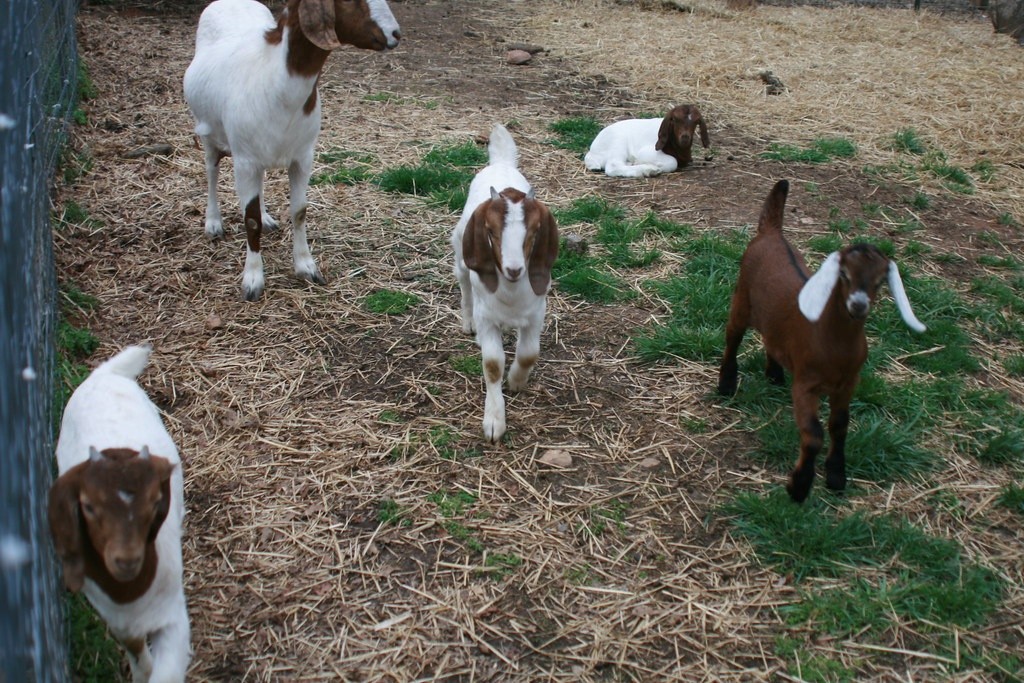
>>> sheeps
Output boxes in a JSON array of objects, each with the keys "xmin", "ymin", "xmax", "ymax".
[
  {"xmin": 46, "ymin": 345, "xmax": 192, "ymax": 683},
  {"xmin": 584, "ymin": 104, "xmax": 711, "ymax": 180},
  {"xmin": 719, "ymin": 180, "xmax": 927, "ymax": 503},
  {"xmin": 181, "ymin": 0, "xmax": 402, "ymax": 302},
  {"xmin": 451, "ymin": 123, "xmax": 559, "ymax": 443}
]
